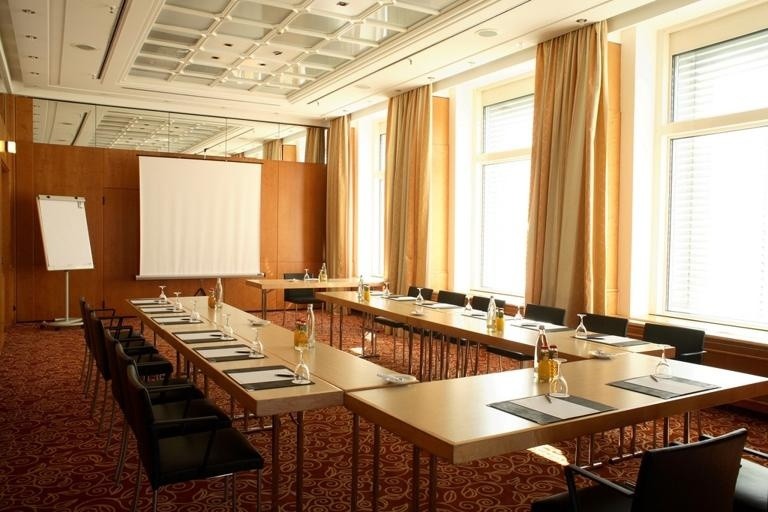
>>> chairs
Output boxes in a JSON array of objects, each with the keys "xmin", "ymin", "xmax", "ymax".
[
  {"xmin": 284, "ymin": 273, "xmax": 325, "ymax": 329},
  {"xmin": 99, "ymin": 342, "xmax": 158, "ymax": 437},
  {"xmin": 579, "ymin": 313, "xmax": 628, "ymax": 338},
  {"xmin": 86, "ymin": 310, "xmax": 123, "ymax": 396},
  {"xmin": 487, "ymin": 303, "xmax": 566, "ymax": 379},
  {"xmin": 378, "ymin": 286, "xmax": 432, "ymax": 368},
  {"xmin": 106, "ymin": 363, "xmax": 185, "ymax": 480},
  {"xmin": 92, "ymin": 321, "xmax": 132, "ymax": 418},
  {"xmin": 439, "ymin": 295, "xmax": 504, "ymax": 375},
  {"xmin": 404, "ymin": 291, "xmax": 466, "ymax": 364},
  {"xmin": 79, "ymin": 297, "xmax": 116, "ymax": 381},
  {"xmin": 620, "ymin": 323, "xmax": 705, "ymax": 447},
  {"xmin": 92, "ymin": 328, "xmax": 145, "ymax": 416},
  {"xmin": 126, "ymin": 361, "xmax": 266, "ymax": 512},
  {"xmin": 533, "ymin": 428, "xmax": 746, "ymax": 511},
  {"xmin": 114, "ymin": 367, "xmax": 206, "ymax": 487}
]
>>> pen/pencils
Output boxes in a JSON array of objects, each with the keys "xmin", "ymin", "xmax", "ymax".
[
  {"xmin": 235, "ymin": 350, "xmax": 250, "ymax": 353},
  {"xmin": 210, "ymin": 334, "xmax": 222, "ymax": 337},
  {"xmin": 545, "ymin": 394, "xmax": 552, "ymax": 404},
  {"xmin": 650, "ymin": 374, "xmax": 658, "ymax": 382},
  {"xmin": 588, "ymin": 337, "xmax": 604, "ymax": 340},
  {"xmin": 181, "ymin": 319, "xmax": 191, "ymax": 320},
  {"xmin": 276, "ymin": 374, "xmax": 294, "ymax": 378},
  {"xmin": 472, "ymin": 314, "xmax": 484, "ymax": 316},
  {"xmin": 521, "ymin": 324, "xmax": 536, "ymax": 327}
]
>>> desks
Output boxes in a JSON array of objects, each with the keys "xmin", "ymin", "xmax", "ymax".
[
  {"xmin": 248, "ymin": 279, "xmax": 379, "ymax": 331},
  {"xmin": 125, "ymin": 295, "xmax": 419, "ymax": 512},
  {"xmin": 345, "ymin": 352, "xmax": 768, "ymax": 511},
  {"xmin": 315, "ymin": 290, "xmax": 676, "ymax": 451}
]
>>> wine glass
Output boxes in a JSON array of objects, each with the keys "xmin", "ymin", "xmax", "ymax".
[
  {"xmin": 158, "ymin": 286, "xmax": 168, "ymax": 305},
  {"xmin": 303, "ymin": 268, "xmax": 309, "ymax": 281},
  {"xmin": 655, "ymin": 344, "xmax": 672, "ymax": 380},
  {"xmin": 190, "ymin": 299, "xmax": 201, "ymax": 323},
  {"xmin": 382, "ymin": 282, "xmax": 390, "ymax": 297},
  {"xmin": 292, "ymin": 343, "xmax": 310, "ymax": 382},
  {"xmin": 220, "ymin": 313, "xmax": 234, "ymax": 340},
  {"xmin": 513, "ymin": 302, "xmax": 523, "ymax": 325},
  {"xmin": 173, "ymin": 292, "xmax": 182, "ymax": 311},
  {"xmin": 575, "ymin": 313, "xmax": 588, "ymax": 336},
  {"xmin": 415, "ymin": 287, "xmax": 423, "ymax": 305},
  {"xmin": 249, "ymin": 321, "xmax": 264, "ymax": 358},
  {"xmin": 463, "ymin": 295, "xmax": 474, "ymax": 310},
  {"xmin": 548, "ymin": 357, "xmax": 568, "ymax": 399}
]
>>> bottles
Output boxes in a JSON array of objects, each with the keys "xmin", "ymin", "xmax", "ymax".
[
  {"xmin": 533, "ymin": 325, "xmax": 547, "ymax": 371},
  {"xmin": 299, "ymin": 318, "xmax": 308, "ymax": 348},
  {"xmin": 537, "ymin": 345, "xmax": 550, "ymax": 385},
  {"xmin": 486, "ymin": 295, "xmax": 497, "ymax": 326},
  {"xmin": 362, "ymin": 284, "xmax": 370, "ymax": 303},
  {"xmin": 546, "ymin": 344, "xmax": 559, "ymax": 377},
  {"xmin": 294, "ymin": 318, "xmax": 300, "ymax": 344},
  {"xmin": 496, "ymin": 308, "xmax": 504, "ymax": 331},
  {"xmin": 214, "ymin": 277, "xmax": 223, "ymax": 309},
  {"xmin": 304, "ymin": 304, "xmax": 315, "ymax": 346},
  {"xmin": 207, "ymin": 288, "xmax": 216, "ymax": 309},
  {"xmin": 358, "ymin": 272, "xmax": 364, "ymax": 302},
  {"xmin": 322, "ymin": 262, "xmax": 328, "ymax": 281}
]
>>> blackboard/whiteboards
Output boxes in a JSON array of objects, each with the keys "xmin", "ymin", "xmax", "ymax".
[{"xmin": 36, "ymin": 194, "xmax": 94, "ymax": 271}]
